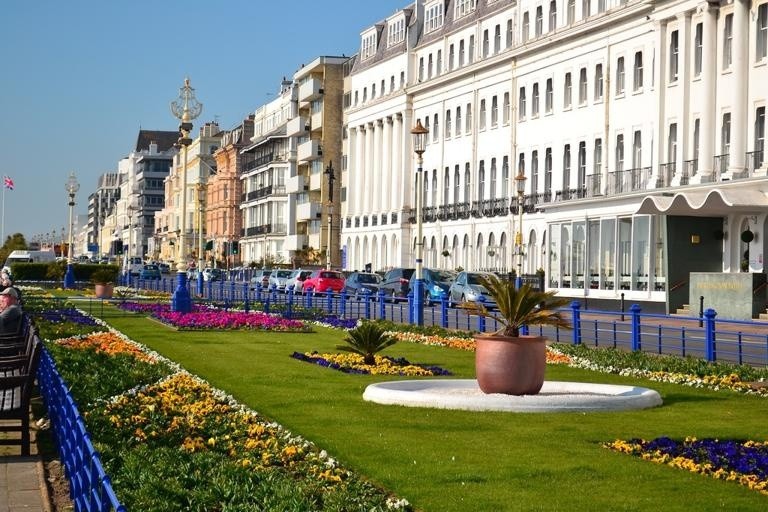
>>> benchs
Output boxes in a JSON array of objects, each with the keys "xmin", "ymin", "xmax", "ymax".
[{"xmin": 1, "ymin": 305, "xmax": 44, "ymax": 457}]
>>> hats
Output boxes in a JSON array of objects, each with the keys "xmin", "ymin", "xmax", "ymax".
[{"xmin": 0, "ymin": 287, "xmax": 19, "ymax": 299}]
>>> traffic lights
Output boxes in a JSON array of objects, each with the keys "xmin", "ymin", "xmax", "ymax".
[
  {"xmin": 232, "ymin": 240, "xmax": 239, "ymax": 254},
  {"xmin": 115, "ymin": 239, "xmax": 123, "ymax": 255}
]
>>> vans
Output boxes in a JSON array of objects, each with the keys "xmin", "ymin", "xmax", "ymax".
[
  {"xmin": 1, "ymin": 248, "xmax": 58, "ymax": 275},
  {"xmin": 121, "ymin": 255, "xmax": 143, "ymax": 277}
]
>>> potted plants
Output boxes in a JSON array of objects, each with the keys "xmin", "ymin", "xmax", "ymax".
[
  {"xmin": 92, "ymin": 268, "xmax": 118, "ymax": 297},
  {"xmin": 455, "ymin": 267, "xmax": 575, "ymax": 394}
]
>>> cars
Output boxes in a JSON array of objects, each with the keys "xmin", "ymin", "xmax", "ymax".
[
  {"xmin": 248, "ymin": 268, "xmax": 507, "ymax": 310},
  {"xmin": 138, "ymin": 264, "xmax": 162, "ymax": 280}
]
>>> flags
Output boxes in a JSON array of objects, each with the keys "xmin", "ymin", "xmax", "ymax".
[{"xmin": 4, "ymin": 176, "xmax": 15, "ymax": 191}]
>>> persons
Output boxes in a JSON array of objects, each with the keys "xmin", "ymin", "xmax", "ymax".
[
  {"xmin": 0, "ymin": 267, "xmax": 22, "ymax": 332},
  {"xmin": 187, "ymin": 259, "xmax": 196, "ymax": 280}
]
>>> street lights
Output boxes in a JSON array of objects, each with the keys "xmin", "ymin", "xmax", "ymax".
[
  {"xmin": 167, "ymin": 74, "xmax": 204, "ymax": 313},
  {"xmin": 514, "ymin": 172, "xmax": 528, "ymax": 288},
  {"xmin": 63, "ymin": 171, "xmax": 81, "ymax": 289},
  {"xmin": 325, "ymin": 199, "xmax": 334, "ymax": 271},
  {"xmin": 194, "ymin": 182, "xmax": 209, "ymax": 297},
  {"xmin": 411, "ymin": 118, "xmax": 429, "ymax": 325}
]
